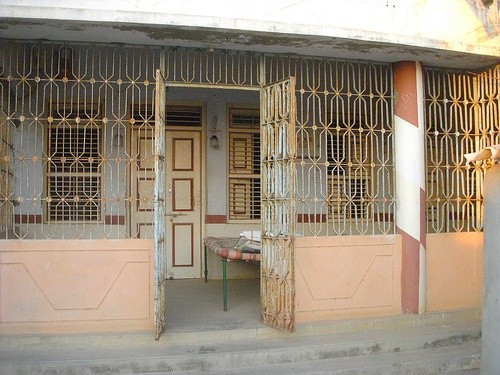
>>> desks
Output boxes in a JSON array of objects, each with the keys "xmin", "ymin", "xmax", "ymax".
[{"xmin": 204, "ymin": 235, "xmax": 263, "ymax": 311}]
